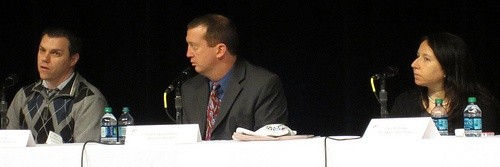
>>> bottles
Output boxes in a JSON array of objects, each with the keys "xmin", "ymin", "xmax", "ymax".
[
  {"xmin": 431, "ymin": 98, "xmax": 448, "ymax": 136},
  {"xmin": 100, "ymin": 107, "xmax": 117, "ymax": 145},
  {"xmin": 463, "ymin": 97, "xmax": 483, "ymax": 138},
  {"xmin": 117, "ymin": 106, "xmax": 135, "ymax": 145}
]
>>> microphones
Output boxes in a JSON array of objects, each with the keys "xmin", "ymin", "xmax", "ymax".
[
  {"xmin": 165, "ymin": 67, "xmax": 192, "ymax": 95},
  {"xmin": 371, "ymin": 65, "xmax": 402, "ymax": 80},
  {"xmin": 1, "ymin": 73, "xmax": 20, "ymax": 92}
]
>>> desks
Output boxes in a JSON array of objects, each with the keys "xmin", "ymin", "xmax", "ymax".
[{"xmin": 0, "ymin": 138, "xmax": 500, "ymax": 167}]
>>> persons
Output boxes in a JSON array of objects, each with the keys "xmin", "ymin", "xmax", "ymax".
[
  {"xmin": 181, "ymin": 14, "xmax": 288, "ymax": 141},
  {"xmin": 7, "ymin": 28, "xmax": 108, "ymax": 144},
  {"xmin": 390, "ymin": 32, "xmax": 498, "ymax": 135}
]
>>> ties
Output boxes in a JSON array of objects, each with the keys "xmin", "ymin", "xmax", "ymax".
[{"xmin": 204, "ymin": 81, "xmax": 221, "ymax": 140}]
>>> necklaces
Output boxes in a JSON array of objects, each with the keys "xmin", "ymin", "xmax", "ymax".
[{"xmin": 428, "ymin": 95, "xmax": 448, "ymax": 106}]
